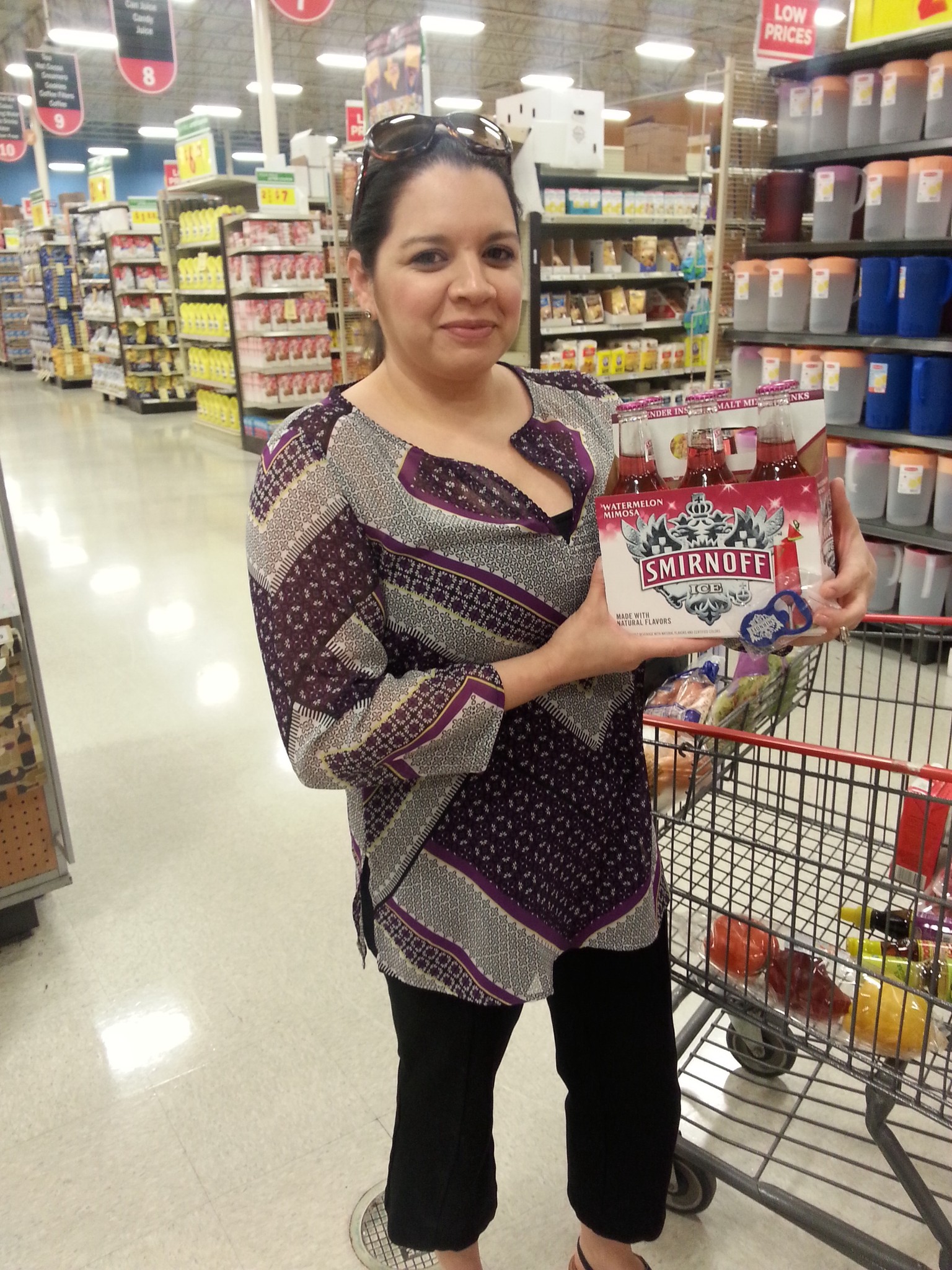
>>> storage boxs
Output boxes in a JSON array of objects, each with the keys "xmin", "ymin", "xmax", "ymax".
[
  {"xmin": 594, "ymin": 391, "xmax": 841, "ymax": 638},
  {"xmin": 625, "ymin": 116, "xmax": 689, "ymax": 174},
  {"xmin": 289, "ymin": 129, "xmax": 333, "ymax": 198},
  {"xmin": 496, "ymin": 87, "xmax": 603, "ymax": 171},
  {"xmin": 59, "ymin": 193, "xmax": 86, "ymax": 214}
]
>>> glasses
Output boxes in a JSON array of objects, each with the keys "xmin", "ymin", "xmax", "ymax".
[{"xmin": 350, "ymin": 112, "xmax": 514, "ymax": 222}]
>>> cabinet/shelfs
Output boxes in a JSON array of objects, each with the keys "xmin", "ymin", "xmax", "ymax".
[
  {"xmin": 0, "ymin": 175, "xmax": 380, "ymax": 456},
  {"xmin": 498, "ymin": 29, "xmax": 952, "ymax": 664}
]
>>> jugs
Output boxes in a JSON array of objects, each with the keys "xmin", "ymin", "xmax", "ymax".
[
  {"xmin": 729, "ymin": 255, "xmax": 951, "ymax": 338},
  {"xmin": 864, "ymin": 538, "xmax": 903, "ymax": 613},
  {"xmin": 730, "ymin": 342, "xmax": 952, "ymax": 435},
  {"xmin": 826, "ymin": 438, "xmax": 952, "ymax": 534},
  {"xmin": 811, "ymin": 165, "xmax": 867, "ymax": 243},
  {"xmin": 897, "ymin": 543, "xmax": 952, "ymax": 618},
  {"xmin": 903, "ymin": 155, "xmax": 952, "ymax": 239},
  {"xmin": 861, "ymin": 160, "xmax": 908, "ymax": 243},
  {"xmin": 777, "ymin": 51, "xmax": 952, "ymax": 156},
  {"xmin": 752, "ymin": 170, "xmax": 808, "ymax": 245}
]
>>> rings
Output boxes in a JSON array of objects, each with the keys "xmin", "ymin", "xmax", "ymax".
[{"xmin": 835, "ymin": 626, "xmax": 850, "ymax": 642}]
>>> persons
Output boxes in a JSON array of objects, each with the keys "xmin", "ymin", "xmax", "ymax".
[{"xmin": 246, "ymin": 126, "xmax": 876, "ymax": 1269}]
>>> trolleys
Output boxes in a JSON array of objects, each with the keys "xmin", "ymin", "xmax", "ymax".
[{"xmin": 639, "ymin": 612, "xmax": 952, "ymax": 1270}]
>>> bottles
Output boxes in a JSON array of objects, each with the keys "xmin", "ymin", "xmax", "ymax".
[
  {"xmin": 747, "ymin": 379, "xmax": 809, "ymax": 481},
  {"xmin": 840, "ymin": 905, "xmax": 952, "ymax": 943},
  {"xmin": 857, "ymin": 951, "xmax": 952, "ymax": 1004},
  {"xmin": 846, "ymin": 935, "xmax": 952, "ymax": 963},
  {"xmin": 677, "ymin": 387, "xmax": 742, "ymax": 486},
  {"xmin": 612, "ymin": 393, "xmax": 671, "ymax": 499}
]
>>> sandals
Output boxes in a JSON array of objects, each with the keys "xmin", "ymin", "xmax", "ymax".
[{"xmin": 569, "ymin": 1235, "xmax": 649, "ymax": 1270}]
